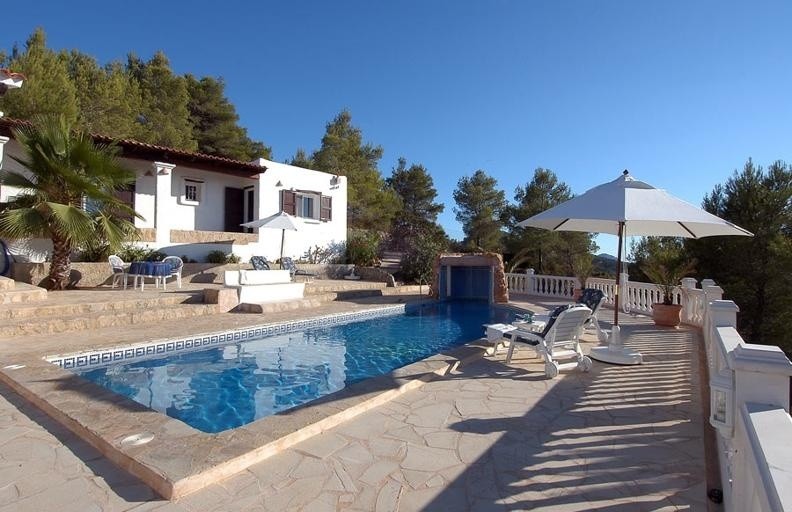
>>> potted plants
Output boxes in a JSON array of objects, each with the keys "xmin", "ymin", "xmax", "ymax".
[
  {"xmin": 571, "ymin": 251, "xmax": 595, "ymax": 302},
  {"xmin": 637, "ymin": 236, "xmax": 698, "ymax": 326}
]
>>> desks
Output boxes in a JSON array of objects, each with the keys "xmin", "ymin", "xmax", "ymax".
[{"xmin": 129, "ymin": 262, "xmax": 172, "ymax": 291}]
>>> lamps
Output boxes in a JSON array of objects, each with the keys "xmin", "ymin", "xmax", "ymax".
[
  {"xmin": 159, "ymin": 167, "xmax": 169, "ymax": 174},
  {"xmin": 143, "ymin": 169, "xmax": 154, "ymax": 176}
]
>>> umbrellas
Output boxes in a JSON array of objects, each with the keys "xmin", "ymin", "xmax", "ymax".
[
  {"xmin": 511, "ymin": 168, "xmax": 758, "ymax": 325},
  {"xmin": 239, "ymin": 209, "xmax": 324, "ymax": 270}
]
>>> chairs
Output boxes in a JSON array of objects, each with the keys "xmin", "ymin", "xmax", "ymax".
[
  {"xmin": 482, "ymin": 288, "xmax": 608, "ymax": 378},
  {"xmin": 251, "ymin": 256, "xmax": 319, "ymax": 282},
  {"xmin": 158, "ymin": 256, "xmax": 184, "ymax": 288},
  {"xmin": 108, "ymin": 255, "xmax": 131, "ymax": 290}
]
